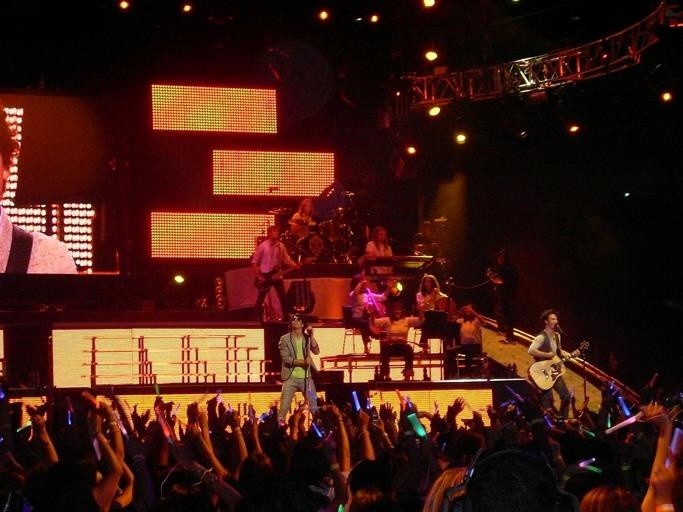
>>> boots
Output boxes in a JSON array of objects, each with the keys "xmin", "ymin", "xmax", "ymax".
[{"xmin": 364, "ymin": 342, "xmax": 369, "ymax": 352}]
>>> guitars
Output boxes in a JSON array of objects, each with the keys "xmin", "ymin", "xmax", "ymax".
[
  {"xmin": 526, "ymin": 339, "xmax": 590, "ymax": 392},
  {"xmin": 253, "ymin": 256, "xmax": 316, "ymax": 293}
]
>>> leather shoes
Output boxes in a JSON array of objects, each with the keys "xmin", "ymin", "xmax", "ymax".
[
  {"xmin": 497, "ymin": 331, "xmax": 504, "ymax": 335},
  {"xmin": 500, "ymin": 337, "xmax": 516, "ymax": 345},
  {"xmin": 381, "ymin": 375, "xmax": 413, "ymax": 380}
]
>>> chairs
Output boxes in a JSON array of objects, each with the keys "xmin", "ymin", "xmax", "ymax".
[{"xmin": 342, "ymin": 306, "xmax": 490, "ymax": 381}]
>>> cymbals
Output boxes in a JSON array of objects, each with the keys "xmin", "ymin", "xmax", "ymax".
[
  {"xmin": 269, "ymin": 206, "xmax": 296, "ymax": 215},
  {"xmin": 340, "ymin": 190, "xmax": 356, "ymax": 196}
]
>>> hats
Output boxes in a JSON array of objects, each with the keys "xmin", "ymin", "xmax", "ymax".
[
  {"xmin": 429, "ymin": 428, "xmax": 474, "ymax": 458},
  {"xmin": 495, "ymin": 248, "xmax": 512, "ymax": 256},
  {"xmin": 466, "ymin": 451, "xmax": 577, "ymax": 511}
]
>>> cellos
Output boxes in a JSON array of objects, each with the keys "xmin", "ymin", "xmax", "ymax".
[{"xmin": 363, "ymin": 290, "xmax": 391, "ymax": 335}]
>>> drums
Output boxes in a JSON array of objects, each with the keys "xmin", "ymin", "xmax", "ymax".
[
  {"xmin": 327, "ymin": 219, "xmax": 341, "ymax": 241},
  {"xmin": 257, "ymin": 235, "xmax": 266, "ymax": 246},
  {"xmin": 338, "ymin": 223, "xmax": 350, "ymax": 245},
  {"xmin": 306, "ymin": 234, "xmax": 332, "ymax": 260},
  {"xmin": 281, "ymin": 231, "xmax": 295, "ymax": 245}
]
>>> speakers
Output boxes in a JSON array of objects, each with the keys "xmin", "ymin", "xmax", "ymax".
[
  {"xmin": 46, "ymin": 387, "xmax": 98, "ymax": 443},
  {"xmin": 325, "ymin": 382, "xmax": 368, "ymax": 410},
  {"xmin": 490, "ymin": 382, "xmax": 554, "ymax": 422}
]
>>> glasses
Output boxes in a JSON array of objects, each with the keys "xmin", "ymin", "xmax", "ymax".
[{"xmin": 292, "ymin": 318, "xmax": 302, "ymax": 321}]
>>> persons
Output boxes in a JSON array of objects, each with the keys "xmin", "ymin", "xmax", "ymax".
[
  {"xmin": 290, "ymin": 196, "xmax": 317, "ymax": 262},
  {"xmin": 526, "ymin": 307, "xmax": 583, "ymax": 422},
  {"xmin": 348, "ymin": 216, "xmax": 481, "ymax": 347},
  {"xmin": 3, "ymin": 372, "xmax": 681, "ymax": 512},
  {"xmin": 1, "ymin": 104, "xmax": 79, "ymax": 276},
  {"xmin": 278, "ymin": 309, "xmax": 320, "ymax": 422},
  {"xmin": 251, "ymin": 224, "xmax": 303, "ymax": 321},
  {"xmin": 598, "ymin": 349, "xmax": 618, "ymax": 371},
  {"xmin": 484, "ymin": 247, "xmax": 517, "ymax": 347}
]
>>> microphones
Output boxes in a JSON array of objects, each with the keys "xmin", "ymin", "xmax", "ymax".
[
  {"xmin": 554, "ymin": 324, "xmax": 563, "ymax": 334},
  {"xmin": 306, "ymin": 324, "xmax": 311, "ymax": 335}
]
